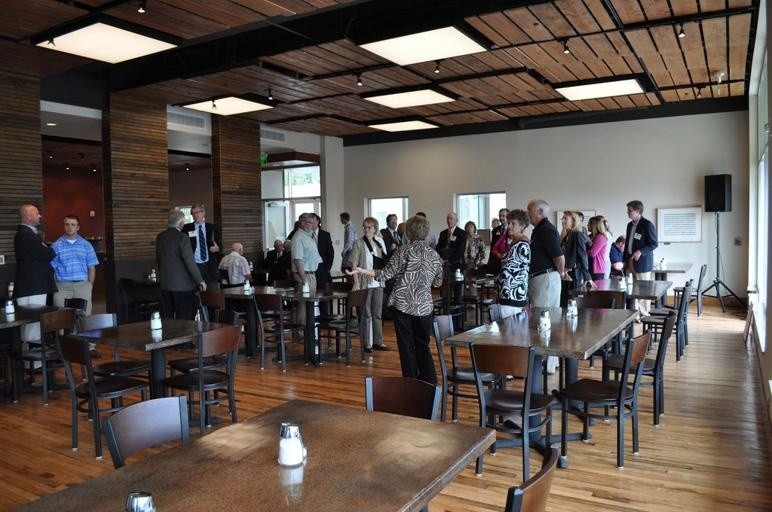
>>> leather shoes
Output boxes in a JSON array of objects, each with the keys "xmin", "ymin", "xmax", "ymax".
[
  {"xmin": 89, "ymin": 349, "xmax": 101, "ymax": 358},
  {"xmin": 364, "ymin": 344, "xmax": 392, "ymax": 353},
  {"xmin": 175, "ymin": 342, "xmax": 196, "ymax": 351},
  {"xmin": 27, "ymin": 366, "xmax": 53, "ymax": 374}
]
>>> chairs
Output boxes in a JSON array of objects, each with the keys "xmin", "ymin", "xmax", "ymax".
[{"xmin": 508, "ymin": 450, "xmax": 557, "ymax": 512}]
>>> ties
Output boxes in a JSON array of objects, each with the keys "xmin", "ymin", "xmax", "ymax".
[
  {"xmin": 312, "ymin": 232, "xmax": 317, "ymax": 241},
  {"xmin": 199, "ymin": 225, "xmax": 207, "ymax": 261},
  {"xmin": 278, "ymin": 253, "xmax": 281, "ymax": 257},
  {"xmin": 448, "ymin": 230, "xmax": 451, "ymax": 246}
]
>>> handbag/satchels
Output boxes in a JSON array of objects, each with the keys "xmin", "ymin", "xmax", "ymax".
[{"xmin": 373, "ymin": 251, "xmax": 390, "ymax": 269}]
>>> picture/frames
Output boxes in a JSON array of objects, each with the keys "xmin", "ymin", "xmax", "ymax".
[
  {"xmin": 556, "ymin": 209, "xmax": 596, "ymax": 236},
  {"xmin": 654, "ymin": 205, "xmax": 702, "ymax": 243}
]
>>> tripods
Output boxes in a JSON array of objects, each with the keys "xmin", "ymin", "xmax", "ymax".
[{"xmin": 688, "ymin": 215, "xmax": 746, "ymax": 313}]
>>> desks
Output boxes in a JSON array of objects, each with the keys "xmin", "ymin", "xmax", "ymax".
[{"xmin": 6, "ymin": 398, "xmax": 495, "ymax": 512}]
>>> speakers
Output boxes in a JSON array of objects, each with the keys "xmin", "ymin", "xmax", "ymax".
[{"xmin": 704, "ymin": 173, "xmax": 732, "ymax": 214}]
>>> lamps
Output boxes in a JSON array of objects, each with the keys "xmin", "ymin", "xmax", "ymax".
[
  {"xmin": 357, "ymin": 73, "xmax": 363, "ymax": 87},
  {"xmin": 677, "ymin": 24, "xmax": 686, "ymax": 38},
  {"xmin": 434, "ymin": 62, "xmax": 440, "ymax": 74},
  {"xmin": 268, "ymin": 90, "xmax": 273, "ymax": 100},
  {"xmin": 562, "ymin": 39, "xmax": 570, "ymax": 54}
]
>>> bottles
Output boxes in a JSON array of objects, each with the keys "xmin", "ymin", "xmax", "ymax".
[
  {"xmin": 151, "ymin": 269, "xmax": 156, "ymax": 278},
  {"xmin": 5, "ymin": 300, "xmax": 15, "ymax": 313},
  {"xmin": 277, "ymin": 421, "xmax": 308, "ymax": 467},
  {"xmin": 249, "ymin": 261, "xmax": 254, "ymax": 271},
  {"xmin": 150, "ymin": 311, "xmax": 162, "ymax": 330},
  {"xmin": 8, "ymin": 282, "xmax": 14, "ymax": 291},
  {"xmin": 243, "ymin": 279, "xmax": 251, "ymax": 291}
]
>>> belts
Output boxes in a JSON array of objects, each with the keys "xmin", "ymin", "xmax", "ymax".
[
  {"xmin": 293, "ymin": 271, "xmax": 315, "ymax": 275},
  {"xmin": 528, "ymin": 268, "xmax": 557, "ymax": 278},
  {"xmin": 196, "ymin": 262, "xmax": 209, "ymax": 266}
]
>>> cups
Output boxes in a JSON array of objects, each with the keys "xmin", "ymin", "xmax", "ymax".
[
  {"xmin": 539, "ymin": 311, "xmax": 552, "ymax": 332},
  {"xmin": 617, "ymin": 277, "xmax": 631, "ymax": 292},
  {"xmin": 126, "ymin": 492, "xmax": 156, "ymax": 512},
  {"xmin": 568, "ymin": 300, "xmax": 579, "ymax": 319},
  {"xmin": 303, "ymin": 283, "xmax": 310, "ymax": 296},
  {"xmin": 623, "ymin": 274, "xmax": 634, "ymax": 288}
]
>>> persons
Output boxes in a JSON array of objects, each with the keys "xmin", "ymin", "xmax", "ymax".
[
  {"xmin": 217, "ymin": 243, "xmax": 253, "ymax": 287},
  {"xmin": 10, "ymin": 204, "xmax": 59, "ymax": 374},
  {"xmin": 181, "ymin": 204, "xmax": 222, "ymax": 281},
  {"xmin": 49, "ymin": 214, "xmax": 100, "ymax": 335},
  {"xmin": 156, "ymin": 211, "xmax": 208, "ymax": 320},
  {"xmin": 435, "ymin": 197, "xmax": 658, "ymax": 378},
  {"xmin": 262, "ymin": 212, "xmax": 444, "ymax": 385}
]
